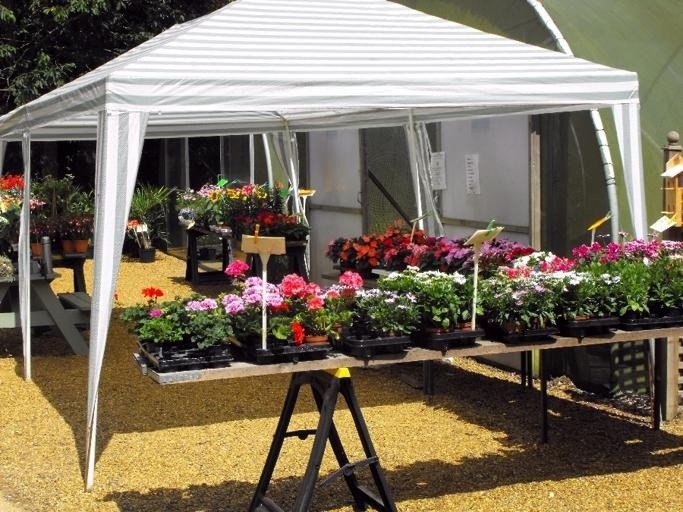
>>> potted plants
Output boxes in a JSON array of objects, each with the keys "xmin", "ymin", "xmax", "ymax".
[{"xmin": 0, "ymin": 171, "xmax": 95, "ymax": 255}]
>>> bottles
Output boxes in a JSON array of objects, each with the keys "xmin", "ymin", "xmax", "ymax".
[{"xmin": 40, "ymin": 235, "xmax": 53, "ymax": 274}]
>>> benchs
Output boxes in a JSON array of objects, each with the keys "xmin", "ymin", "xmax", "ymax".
[{"xmin": 57, "ymin": 289, "xmax": 90, "ymax": 355}]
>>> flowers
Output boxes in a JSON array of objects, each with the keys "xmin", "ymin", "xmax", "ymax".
[
  {"xmin": 175, "ymin": 181, "xmax": 313, "ymax": 241},
  {"xmin": 113, "ymin": 218, "xmax": 683, "ymax": 354}
]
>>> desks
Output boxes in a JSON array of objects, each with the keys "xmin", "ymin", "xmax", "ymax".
[
  {"xmin": 134, "ymin": 323, "xmax": 682, "ymax": 509},
  {"xmin": 0, "ymin": 260, "xmax": 88, "ymax": 355}
]
